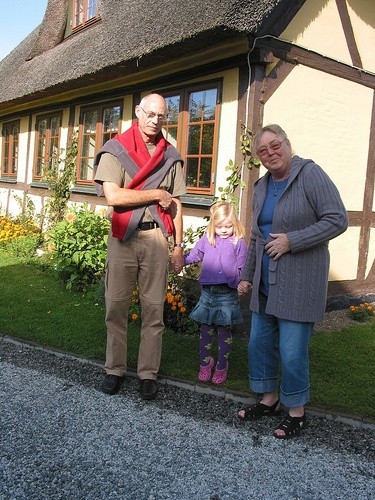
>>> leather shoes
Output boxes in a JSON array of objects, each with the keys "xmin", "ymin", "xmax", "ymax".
[
  {"xmin": 138, "ymin": 378, "xmax": 158, "ymax": 400},
  {"xmin": 101, "ymin": 375, "xmax": 123, "ymax": 394}
]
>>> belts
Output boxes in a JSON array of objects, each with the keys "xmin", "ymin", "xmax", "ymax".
[{"xmin": 135, "ymin": 220, "xmax": 158, "ymax": 231}]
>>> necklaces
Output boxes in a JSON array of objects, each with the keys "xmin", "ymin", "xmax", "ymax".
[{"xmin": 271, "ymin": 168, "xmax": 290, "ymax": 196}]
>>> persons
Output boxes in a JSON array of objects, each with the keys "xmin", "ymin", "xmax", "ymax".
[
  {"xmin": 179, "ymin": 201, "xmax": 254, "ymax": 384},
  {"xmin": 92, "ymin": 94, "xmax": 189, "ymax": 398},
  {"xmin": 235, "ymin": 125, "xmax": 349, "ymax": 439}
]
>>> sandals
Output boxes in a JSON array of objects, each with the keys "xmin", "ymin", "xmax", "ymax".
[
  {"xmin": 238, "ymin": 399, "xmax": 283, "ymax": 420},
  {"xmin": 273, "ymin": 409, "xmax": 307, "ymax": 439}
]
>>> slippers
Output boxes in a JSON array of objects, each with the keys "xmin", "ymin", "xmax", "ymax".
[
  {"xmin": 197, "ymin": 356, "xmax": 215, "ymax": 383},
  {"xmin": 212, "ymin": 360, "xmax": 229, "ymax": 384}
]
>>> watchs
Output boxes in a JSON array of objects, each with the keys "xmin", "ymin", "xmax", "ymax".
[{"xmin": 173, "ymin": 242, "xmax": 186, "ymax": 250}]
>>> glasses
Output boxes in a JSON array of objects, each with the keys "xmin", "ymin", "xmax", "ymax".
[
  {"xmin": 255, "ymin": 139, "xmax": 288, "ymax": 154},
  {"xmin": 139, "ymin": 107, "xmax": 170, "ymax": 122}
]
